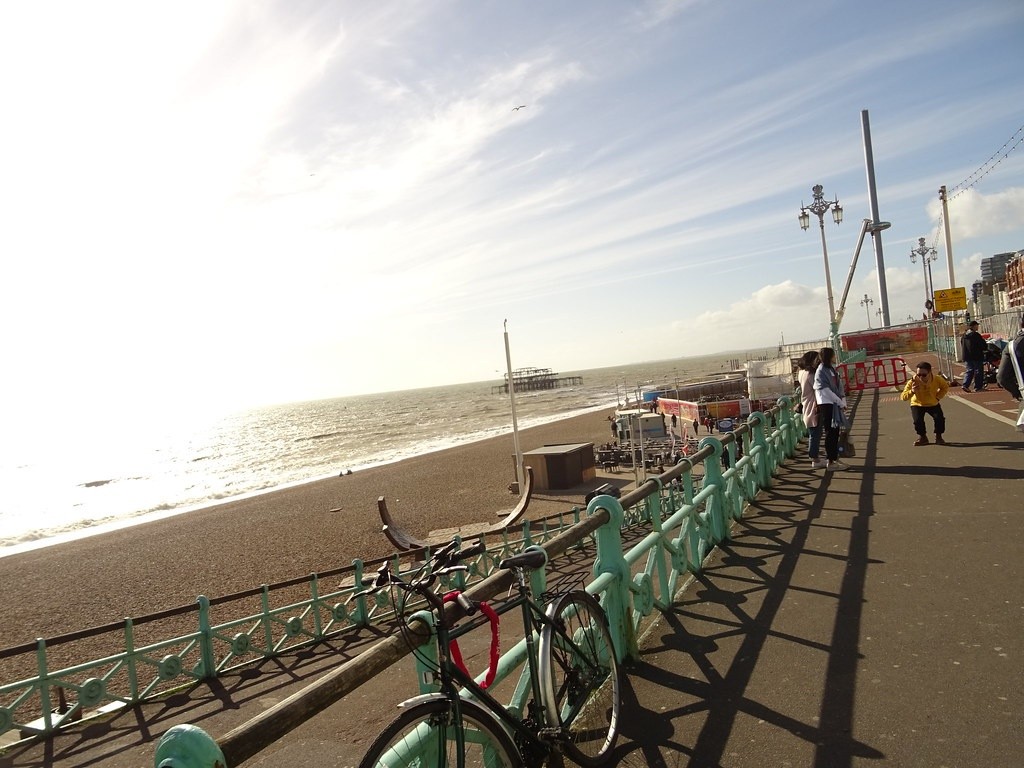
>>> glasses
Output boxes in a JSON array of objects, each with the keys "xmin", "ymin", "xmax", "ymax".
[{"xmin": 917, "ymin": 373, "xmax": 929, "ymax": 377}]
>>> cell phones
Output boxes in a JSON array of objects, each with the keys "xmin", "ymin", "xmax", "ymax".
[{"xmin": 912, "ymin": 379, "xmax": 919, "ymax": 387}]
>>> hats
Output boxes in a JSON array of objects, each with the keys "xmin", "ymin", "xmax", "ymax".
[{"xmin": 970, "ymin": 321, "xmax": 980, "ymax": 326}]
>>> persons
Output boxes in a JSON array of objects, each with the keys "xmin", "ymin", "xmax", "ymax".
[
  {"xmin": 611, "ymin": 422, "xmax": 617, "ymax": 437},
  {"xmin": 900, "ymin": 362, "xmax": 949, "ymax": 446},
  {"xmin": 798, "ymin": 348, "xmax": 850, "ymax": 471},
  {"xmin": 962, "ymin": 319, "xmax": 988, "ymax": 392},
  {"xmin": 650, "ymin": 401, "xmax": 714, "ymax": 434},
  {"xmin": 657, "ymin": 453, "xmax": 685, "ymax": 492},
  {"xmin": 996, "ymin": 313, "xmax": 1024, "ymax": 402},
  {"xmin": 720, "ymin": 434, "xmax": 743, "ymax": 472}
]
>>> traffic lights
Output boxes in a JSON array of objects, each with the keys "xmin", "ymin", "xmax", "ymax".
[
  {"xmin": 971, "ymin": 289, "xmax": 977, "ymax": 303},
  {"xmin": 966, "ymin": 313, "xmax": 970, "ymax": 322}
]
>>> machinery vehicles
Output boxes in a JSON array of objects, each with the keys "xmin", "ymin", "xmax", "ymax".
[{"xmin": 828, "ymin": 219, "xmax": 874, "ymax": 341}]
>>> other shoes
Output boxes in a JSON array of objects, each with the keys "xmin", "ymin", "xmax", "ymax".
[
  {"xmin": 962, "ymin": 386, "xmax": 971, "ymax": 393},
  {"xmin": 811, "ymin": 459, "xmax": 827, "ymax": 467},
  {"xmin": 827, "ymin": 461, "xmax": 847, "ymax": 471},
  {"xmin": 974, "ymin": 387, "xmax": 984, "ymax": 392},
  {"xmin": 1015, "ymin": 424, "xmax": 1024, "ymax": 432},
  {"xmin": 913, "ymin": 437, "xmax": 929, "ymax": 446},
  {"xmin": 936, "ymin": 437, "xmax": 945, "ymax": 444},
  {"xmin": 793, "ymin": 403, "xmax": 802, "ymax": 414},
  {"xmin": 836, "ymin": 460, "xmax": 850, "ymax": 468}
]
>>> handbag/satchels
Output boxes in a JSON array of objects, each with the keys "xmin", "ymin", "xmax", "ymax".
[
  {"xmin": 1017, "ymin": 400, "xmax": 1024, "ymax": 425},
  {"xmin": 838, "ymin": 441, "xmax": 856, "ymax": 457}
]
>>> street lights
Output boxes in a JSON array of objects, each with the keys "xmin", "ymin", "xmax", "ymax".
[
  {"xmin": 615, "ymin": 413, "xmax": 643, "ymax": 489},
  {"xmin": 876, "ymin": 308, "xmax": 882, "ymax": 327},
  {"xmin": 910, "ymin": 237, "xmax": 938, "ymax": 352},
  {"xmin": 798, "ymin": 184, "xmax": 843, "ymax": 363},
  {"xmin": 860, "ymin": 294, "xmax": 874, "ymax": 329},
  {"xmin": 716, "ymin": 395, "xmax": 719, "ymax": 420}
]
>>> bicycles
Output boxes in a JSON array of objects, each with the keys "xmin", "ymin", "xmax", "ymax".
[{"xmin": 358, "ymin": 541, "xmax": 623, "ymax": 768}]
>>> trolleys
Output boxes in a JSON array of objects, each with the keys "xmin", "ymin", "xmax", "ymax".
[{"xmin": 983, "ymin": 343, "xmax": 1003, "ymax": 388}]
